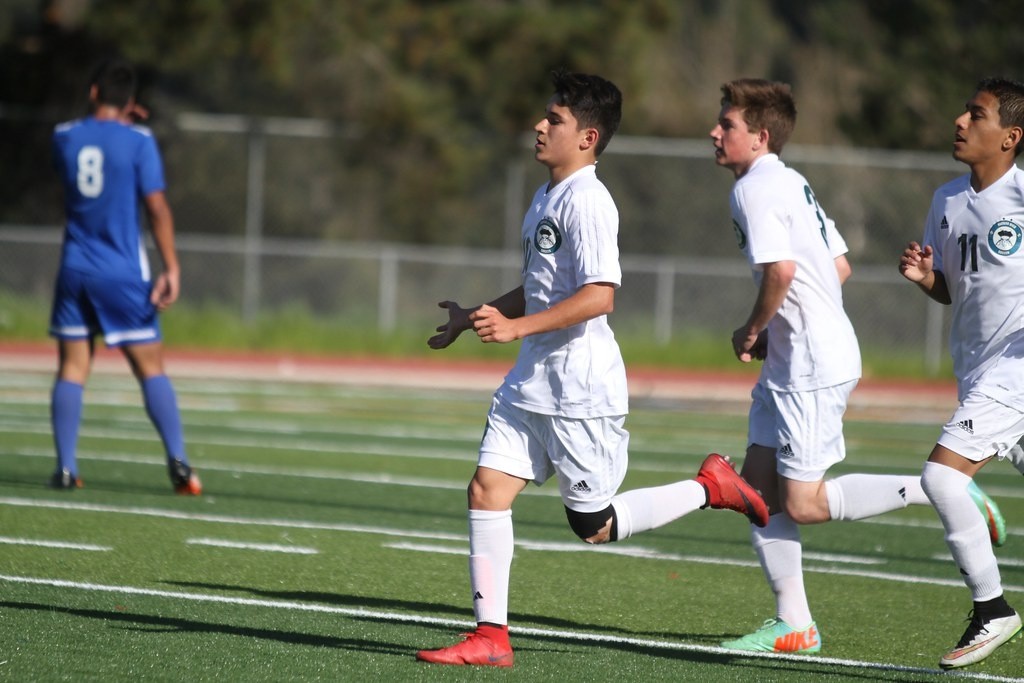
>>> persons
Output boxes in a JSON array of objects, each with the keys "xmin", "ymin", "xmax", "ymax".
[
  {"xmin": 710, "ymin": 77, "xmax": 1008, "ymax": 653},
  {"xmin": 416, "ymin": 72, "xmax": 772, "ymax": 668},
  {"xmin": 899, "ymin": 79, "xmax": 1023, "ymax": 671},
  {"xmin": 48, "ymin": 61, "xmax": 204, "ymax": 495}
]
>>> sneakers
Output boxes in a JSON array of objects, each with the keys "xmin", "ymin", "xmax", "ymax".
[
  {"xmin": 699, "ymin": 452, "xmax": 769, "ymax": 527},
  {"xmin": 718, "ymin": 617, "xmax": 821, "ymax": 654},
  {"xmin": 939, "ymin": 610, "xmax": 1024, "ymax": 671},
  {"xmin": 169, "ymin": 457, "xmax": 203, "ymax": 494},
  {"xmin": 53, "ymin": 468, "xmax": 83, "ymax": 490},
  {"xmin": 965, "ymin": 478, "xmax": 1008, "ymax": 547},
  {"xmin": 415, "ymin": 631, "xmax": 515, "ymax": 667}
]
